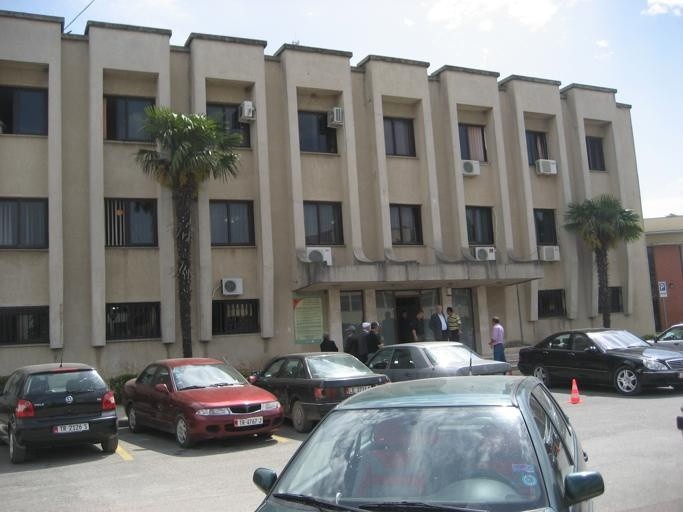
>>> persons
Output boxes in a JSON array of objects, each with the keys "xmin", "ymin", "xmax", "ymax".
[
  {"xmin": 428, "ymin": 303, "xmax": 448, "ymax": 340},
  {"xmin": 320, "ymin": 331, "xmax": 338, "ymax": 352},
  {"xmin": 350, "ymin": 305, "xmax": 424, "ymax": 363},
  {"xmin": 480, "ymin": 432, "xmax": 530, "ymax": 496},
  {"xmin": 487, "ymin": 315, "xmax": 507, "ymax": 363},
  {"xmin": 342, "ymin": 325, "xmax": 355, "ymax": 353},
  {"xmin": 445, "ymin": 306, "xmax": 462, "ymax": 342}
]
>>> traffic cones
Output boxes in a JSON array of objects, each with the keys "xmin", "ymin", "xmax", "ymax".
[{"xmin": 565, "ymin": 378, "xmax": 585, "ymax": 405}]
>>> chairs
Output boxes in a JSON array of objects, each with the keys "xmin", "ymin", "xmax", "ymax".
[{"xmin": 351, "ymin": 420, "xmax": 428, "ymax": 498}]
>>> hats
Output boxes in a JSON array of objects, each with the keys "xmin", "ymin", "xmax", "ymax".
[
  {"xmin": 362, "ymin": 322, "xmax": 369, "ymax": 328},
  {"xmin": 344, "ymin": 325, "xmax": 355, "ymax": 331}
]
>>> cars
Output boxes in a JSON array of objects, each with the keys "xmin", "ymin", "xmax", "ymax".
[
  {"xmin": 247, "ymin": 353, "xmax": 389, "ymax": 434},
  {"xmin": 366, "ymin": 341, "xmax": 511, "ymax": 379},
  {"xmin": 517, "ymin": 329, "xmax": 683, "ymax": 396},
  {"xmin": 124, "ymin": 358, "xmax": 283, "ymax": 450},
  {"xmin": 646, "ymin": 324, "xmax": 682, "ymax": 351},
  {"xmin": 1, "ymin": 364, "xmax": 116, "ymax": 462},
  {"xmin": 255, "ymin": 377, "xmax": 604, "ymax": 512}
]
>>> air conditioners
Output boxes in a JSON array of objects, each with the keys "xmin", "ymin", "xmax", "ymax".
[
  {"xmin": 535, "ymin": 158, "xmax": 558, "ymax": 175},
  {"xmin": 461, "ymin": 160, "xmax": 480, "ymax": 176},
  {"xmin": 222, "ymin": 277, "xmax": 244, "ymax": 296},
  {"xmin": 472, "ymin": 246, "xmax": 497, "ymax": 261},
  {"xmin": 538, "ymin": 245, "xmax": 561, "ymax": 262},
  {"xmin": 306, "ymin": 247, "xmax": 333, "ymax": 267},
  {"xmin": 237, "ymin": 100, "xmax": 257, "ymax": 124},
  {"xmin": 327, "ymin": 107, "xmax": 345, "ymax": 129}
]
